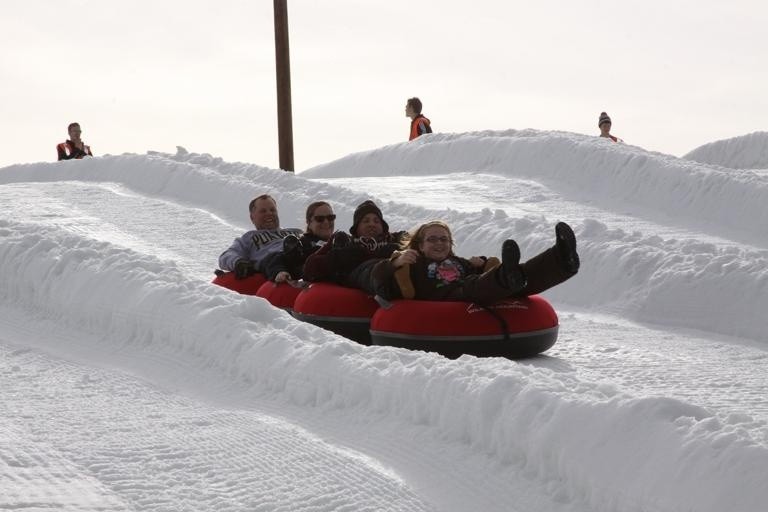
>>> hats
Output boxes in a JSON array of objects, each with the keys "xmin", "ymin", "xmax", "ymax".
[{"xmin": 349, "ymin": 200, "xmax": 389, "ymax": 234}]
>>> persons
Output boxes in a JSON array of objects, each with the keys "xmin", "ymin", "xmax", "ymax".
[
  {"xmin": 218, "ymin": 193, "xmax": 306, "ymax": 281},
  {"xmin": 371, "ymin": 219, "xmax": 582, "ymax": 308},
  {"xmin": 597, "ymin": 110, "xmax": 625, "ymax": 145},
  {"xmin": 302, "ymin": 201, "xmax": 503, "ymax": 300},
  {"xmin": 56, "ymin": 121, "xmax": 93, "ymax": 162},
  {"xmin": 259, "ymin": 201, "xmax": 355, "ymax": 287},
  {"xmin": 404, "ymin": 95, "xmax": 434, "ymax": 141}
]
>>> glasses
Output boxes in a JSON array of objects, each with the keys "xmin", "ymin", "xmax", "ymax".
[
  {"xmin": 314, "ymin": 214, "xmax": 336, "ymax": 222},
  {"xmin": 427, "ymin": 235, "xmax": 448, "ymax": 243}
]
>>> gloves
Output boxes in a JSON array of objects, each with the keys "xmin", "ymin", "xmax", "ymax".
[{"xmin": 235, "ymin": 258, "xmax": 258, "ymax": 279}]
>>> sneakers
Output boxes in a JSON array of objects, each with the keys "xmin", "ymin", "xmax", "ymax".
[
  {"xmin": 554, "ymin": 221, "xmax": 580, "ymax": 273},
  {"xmin": 501, "ymin": 239, "xmax": 528, "ymax": 289},
  {"xmin": 391, "ymin": 256, "xmax": 416, "ymax": 300},
  {"xmin": 484, "ymin": 256, "xmax": 500, "ymax": 272}
]
